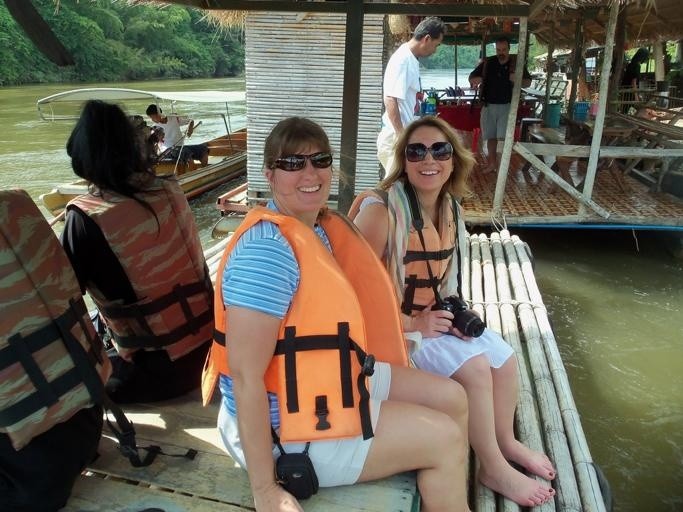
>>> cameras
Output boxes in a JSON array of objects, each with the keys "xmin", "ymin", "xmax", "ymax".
[{"xmin": 431, "ymin": 294, "xmax": 485, "ymax": 338}]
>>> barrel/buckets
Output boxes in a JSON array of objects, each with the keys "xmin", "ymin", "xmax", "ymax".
[{"xmin": 542, "ymin": 104, "xmax": 560, "ymax": 128}]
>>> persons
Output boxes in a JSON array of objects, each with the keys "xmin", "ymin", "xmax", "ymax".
[
  {"xmin": 209, "ymin": 116, "xmax": 477, "ymax": 512},
  {"xmin": 621, "ymin": 47, "xmax": 650, "ymax": 119},
  {"xmin": 58, "ymin": 99, "xmax": 218, "ymax": 407},
  {"xmin": 345, "ymin": 115, "xmax": 561, "ymax": 512},
  {"xmin": 132, "ymin": 115, "xmax": 195, "ymax": 177},
  {"xmin": 467, "ymin": 36, "xmax": 532, "ymax": 174},
  {"xmin": 663, "ymin": 53, "xmax": 673, "ymax": 74},
  {"xmin": 0, "ymin": 187, "xmax": 114, "ymax": 511},
  {"xmin": 145, "ymin": 103, "xmax": 211, "ymax": 171},
  {"xmin": 376, "ymin": 15, "xmax": 448, "ymax": 183}
]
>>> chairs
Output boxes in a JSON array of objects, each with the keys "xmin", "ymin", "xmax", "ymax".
[{"xmin": 446, "ymin": 85, "xmax": 464, "ymax": 96}]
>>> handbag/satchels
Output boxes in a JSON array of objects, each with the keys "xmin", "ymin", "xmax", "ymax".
[{"xmin": 276, "ymin": 442, "xmax": 319, "ymax": 499}]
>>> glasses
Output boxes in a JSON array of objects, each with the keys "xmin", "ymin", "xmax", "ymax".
[
  {"xmin": 268, "ymin": 152, "xmax": 333, "ymax": 171},
  {"xmin": 406, "ymin": 142, "xmax": 453, "ymax": 162}
]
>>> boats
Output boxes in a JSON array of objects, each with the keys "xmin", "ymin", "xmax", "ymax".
[{"xmin": 39, "ymin": 126, "xmax": 246, "ymax": 220}]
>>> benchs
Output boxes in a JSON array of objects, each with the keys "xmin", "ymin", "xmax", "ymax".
[
  {"xmin": 534, "ymin": 78, "xmax": 568, "ymax": 119},
  {"xmin": 606, "ymin": 94, "xmax": 683, "ymax": 193},
  {"xmin": 522, "ymin": 127, "xmax": 584, "ymax": 194}
]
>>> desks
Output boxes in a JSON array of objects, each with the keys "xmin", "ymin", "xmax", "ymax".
[
  {"xmin": 521, "ymin": 86, "xmax": 546, "ymax": 97},
  {"xmin": 551, "ymin": 113, "xmax": 639, "ymax": 197}
]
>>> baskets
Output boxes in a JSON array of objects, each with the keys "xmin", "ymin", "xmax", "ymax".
[{"xmin": 574, "ymin": 102, "xmax": 588, "ymax": 114}]
